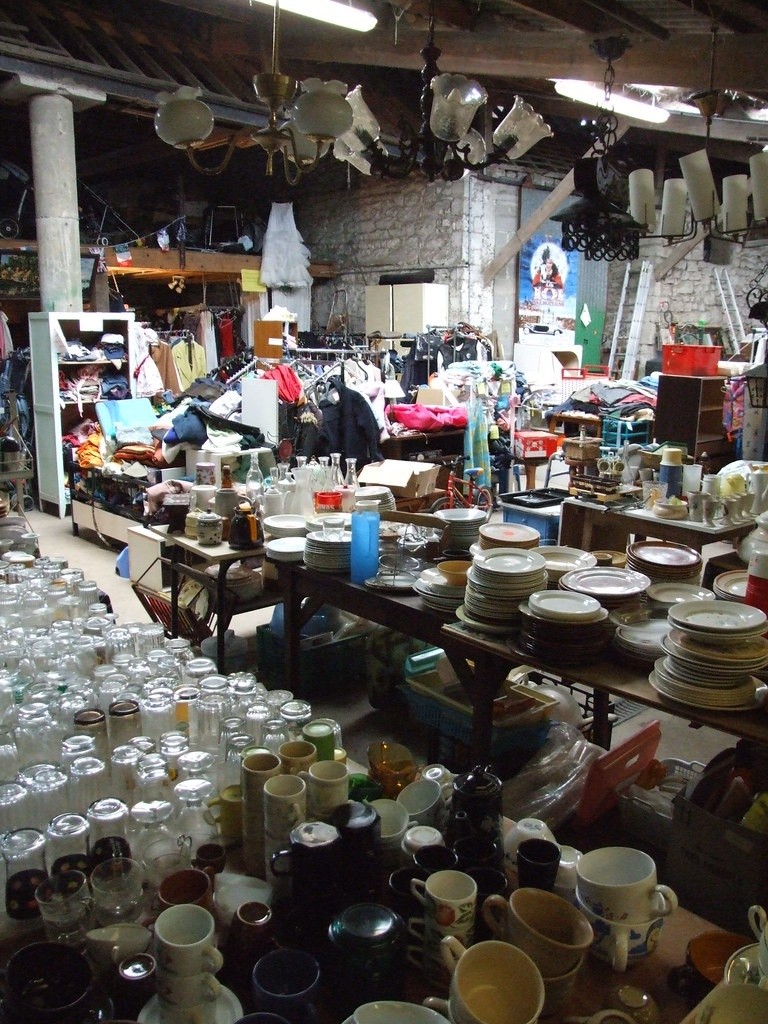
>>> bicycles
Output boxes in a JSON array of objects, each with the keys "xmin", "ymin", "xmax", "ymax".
[{"xmin": 429, "ymin": 454, "xmax": 494, "ymax": 525}]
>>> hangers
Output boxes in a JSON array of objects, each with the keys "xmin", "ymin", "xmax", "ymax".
[
  {"xmin": 297, "ymin": 368, "xmax": 336, "ymax": 407},
  {"xmin": 315, "ymin": 332, "xmax": 365, "ymax": 346},
  {"xmin": 221, "ymin": 351, "xmax": 247, "ymax": 378},
  {"xmin": 445, "ymin": 323, "xmax": 483, "ymax": 342},
  {"xmin": 155, "ymin": 329, "xmax": 194, "ymax": 345},
  {"xmin": 292, "ymin": 347, "xmax": 370, "ymax": 366}
]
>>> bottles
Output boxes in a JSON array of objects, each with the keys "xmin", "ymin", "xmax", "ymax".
[
  {"xmin": 222, "ymin": 452, "xmax": 360, "ymax": 549},
  {"xmin": 658, "ymin": 447, "xmax": 683, "ymax": 497}
]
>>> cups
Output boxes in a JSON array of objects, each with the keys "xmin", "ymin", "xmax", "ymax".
[
  {"xmin": 683, "ymin": 464, "xmax": 768, "ymax": 527},
  {"xmin": 185, "ymin": 462, "xmax": 252, "ymax": 546},
  {"xmin": 316, "ymin": 490, "xmax": 343, "ymax": 513},
  {"xmin": 321, "ymin": 516, "xmax": 345, "ymax": 543},
  {"xmin": 351, "ymin": 511, "xmax": 380, "ymax": 584},
  {"xmin": 0, "ymin": 529, "xmax": 768, "ymax": 1023}
]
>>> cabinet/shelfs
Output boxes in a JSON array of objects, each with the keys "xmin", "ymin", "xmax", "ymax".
[
  {"xmin": 364, "ymin": 282, "xmax": 449, "ymax": 360},
  {"xmin": 378, "ymin": 428, "xmax": 463, "ymax": 494},
  {"xmin": 27, "ymin": 311, "xmax": 136, "ymax": 519},
  {"xmin": 653, "ymin": 373, "xmax": 733, "ymax": 474}
]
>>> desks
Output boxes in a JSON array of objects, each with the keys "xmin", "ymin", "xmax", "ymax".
[
  {"xmin": 263, "ymin": 555, "xmax": 768, "ymax": 849},
  {"xmin": 150, "ymin": 524, "xmax": 284, "ymax": 675},
  {"xmin": 557, "ymin": 496, "xmax": 757, "ymax": 555},
  {"xmin": 674, "ymin": 324, "xmax": 723, "ymax": 346},
  {"xmin": 549, "ymin": 413, "xmax": 602, "ymax": 438}
]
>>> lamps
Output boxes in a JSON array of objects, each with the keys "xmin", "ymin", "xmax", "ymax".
[
  {"xmin": 742, "ymin": 362, "xmax": 768, "ymax": 408},
  {"xmin": 168, "ymin": 280, "xmax": 178, "ymax": 289},
  {"xmin": 175, "ymin": 283, "xmax": 184, "ymax": 293},
  {"xmin": 332, "ymin": 0, "xmax": 555, "ymax": 185},
  {"xmin": 628, "ymin": 25, "xmax": 768, "ymax": 250},
  {"xmin": 153, "ymin": 0, "xmax": 354, "ymax": 186}
]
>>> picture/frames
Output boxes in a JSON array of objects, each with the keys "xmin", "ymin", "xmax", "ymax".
[{"xmin": 0, "ymin": 248, "xmax": 100, "ymax": 304}]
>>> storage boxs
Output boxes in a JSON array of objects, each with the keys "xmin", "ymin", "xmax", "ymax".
[
  {"xmin": 497, "ymin": 495, "xmax": 560, "ymax": 547},
  {"xmin": 514, "ymin": 430, "xmax": 559, "ymax": 459},
  {"xmin": 661, "ymin": 344, "xmax": 724, "ymax": 376},
  {"xmin": 256, "ymin": 623, "xmax": 284, "ymax": 675},
  {"xmin": 291, "ymin": 631, "xmax": 372, "ymax": 675},
  {"xmin": 357, "ymin": 458, "xmax": 441, "ymax": 498},
  {"xmin": 663, "ymin": 786, "xmax": 768, "ymax": 936},
  {"xmin": 404, "ymin": 657, "xmax": 562, "ymax": 728}
]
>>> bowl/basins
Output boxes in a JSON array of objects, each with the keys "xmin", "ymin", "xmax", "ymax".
[{"xmin": 652, "ymin": 498, "xmax": 688, "ymax": 519}]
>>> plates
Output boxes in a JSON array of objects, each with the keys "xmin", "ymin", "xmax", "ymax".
[{"xmin": 263, "ymin": 485, "xmax": 767, "ymax": 712}]
